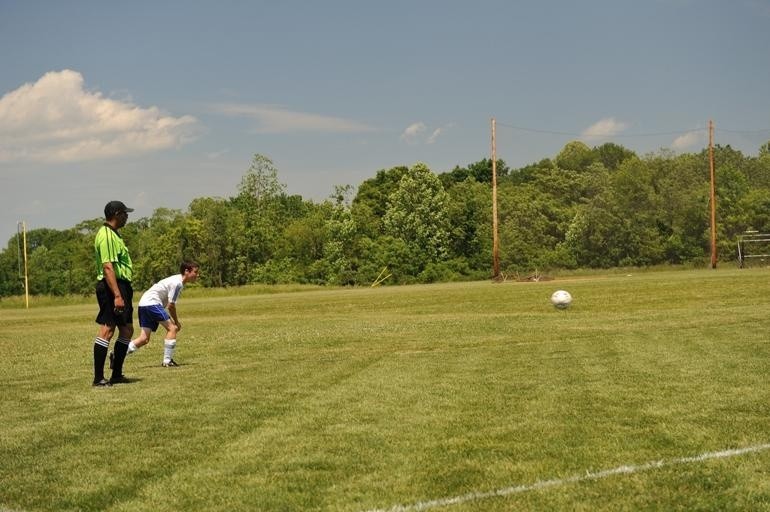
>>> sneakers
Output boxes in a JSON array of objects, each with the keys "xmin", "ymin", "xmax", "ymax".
[
  {"xmin": 162, "ymin": 360, "xmax": 178, "ymax": 368},
  {"xmin": 92, "ymin": 374, "xmax": 131, "ymax": 386},
  {"xmin": 108, "ymin": 350, "xmax": 115, "ymax": 369}
]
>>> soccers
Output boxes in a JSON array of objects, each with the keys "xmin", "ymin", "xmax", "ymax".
[{"xmin": 552, "ymin": 290, "xmax": 573, "ymax": 309}]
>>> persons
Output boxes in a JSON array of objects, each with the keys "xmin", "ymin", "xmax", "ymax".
[
  {"xmin": 107, "ymin": 260, "xmax": 200, "ymax": 368},
  {"xmin": 90, "ymin": 200, "xmax": 136, "ymax": 389}
]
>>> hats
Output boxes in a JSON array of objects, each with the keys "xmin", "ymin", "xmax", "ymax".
[{"xmin": 104, "ymin": 201, "xmax": 135, "ymax": 218}]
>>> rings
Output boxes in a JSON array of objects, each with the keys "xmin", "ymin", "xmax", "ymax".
[{"xmin": 118, "ymin": 310, "xmax": 124, "ymax": 312}]
